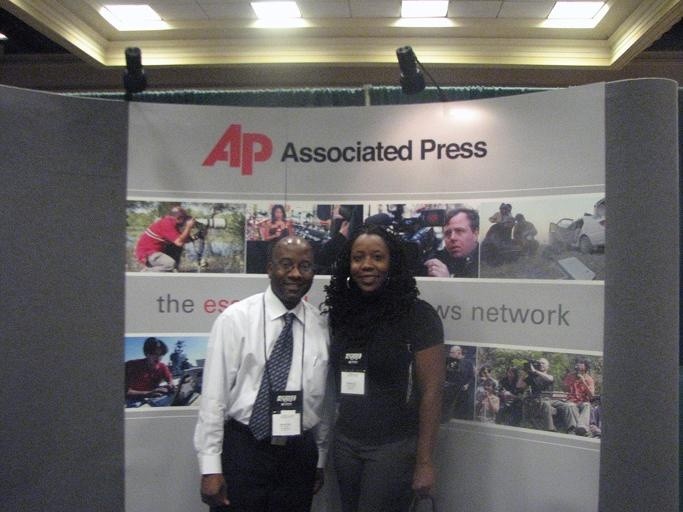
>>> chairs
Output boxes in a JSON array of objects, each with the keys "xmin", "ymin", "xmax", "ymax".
[{"xmin": 567, "ymin": 425, "xmax": 601, "ymax": 438}]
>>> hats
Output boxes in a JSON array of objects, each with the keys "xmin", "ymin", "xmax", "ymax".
[{"xmin": 549, "ymin": 216, "xmax": 605, "ymax": 254}]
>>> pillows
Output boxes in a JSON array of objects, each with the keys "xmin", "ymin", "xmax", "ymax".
[{"xmin": 143, "ymin": 337, "xmax": 170, "ymax": 357}]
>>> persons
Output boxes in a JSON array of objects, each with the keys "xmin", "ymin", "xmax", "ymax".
[
  {"xmin": 191, "ymin": 234, "xmax": 339, "ymax": 512},
  {"xmin": 422, "ymin": 208, "xmax": 480, "ymax": 279},
  {"xmin": 477, "ymin": 354, "xmax": 601, "ymax": 438},
  {"xmin": 446, "ymin": 345, "xmax": 476, "ymax": 420},
  {"xmin": 323, "ymin": 223, "xmax": 447, "ymax": 512},
  {"xmin": 309, "ymin": 206, "xmax": 362, "ymax": 276},
  {"xmin": 487, "ymin": 202, "xmax": 514, "ymax": 242},
  {"xmin": 511, "ymin": 214, "xmax": 539, "ymax": 258},
  {"xmin": 257, "ymin": 204, "xmax": 291, "ymax": 244},
  {"xmin": 124, "ymin": 336, "xmax": 177, "ymax": 408},
  {"xmin": 133, "ymin": 206, "xmax": 194, "ymax": 273}
]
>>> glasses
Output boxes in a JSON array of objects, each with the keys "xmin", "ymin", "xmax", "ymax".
[
  {"xmin": 395, "ymin": 45, "xmax": 448, "ymax": 104},
  {"xmin": 123, "ymin": 43, "xmax": 147, "ymax": 100}
]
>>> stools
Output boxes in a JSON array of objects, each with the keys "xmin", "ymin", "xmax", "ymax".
[{"xmin": 226, "ymin": 416, "xmax": 322, "ymax": 447}]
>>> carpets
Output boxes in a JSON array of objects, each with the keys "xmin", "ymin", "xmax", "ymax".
[{"xmin": 248, "ymin": 312, "xmax": 295, "ymax": 443}]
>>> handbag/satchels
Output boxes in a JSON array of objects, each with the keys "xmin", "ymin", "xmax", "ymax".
[{"xmin": 417, "ymin": 439, "xmax": 436, "ymax": 451}]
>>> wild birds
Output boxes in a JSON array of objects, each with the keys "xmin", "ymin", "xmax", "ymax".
[{"xmin": 147, "ymin": 366, "xmax": 203, "ymax": 407}]
